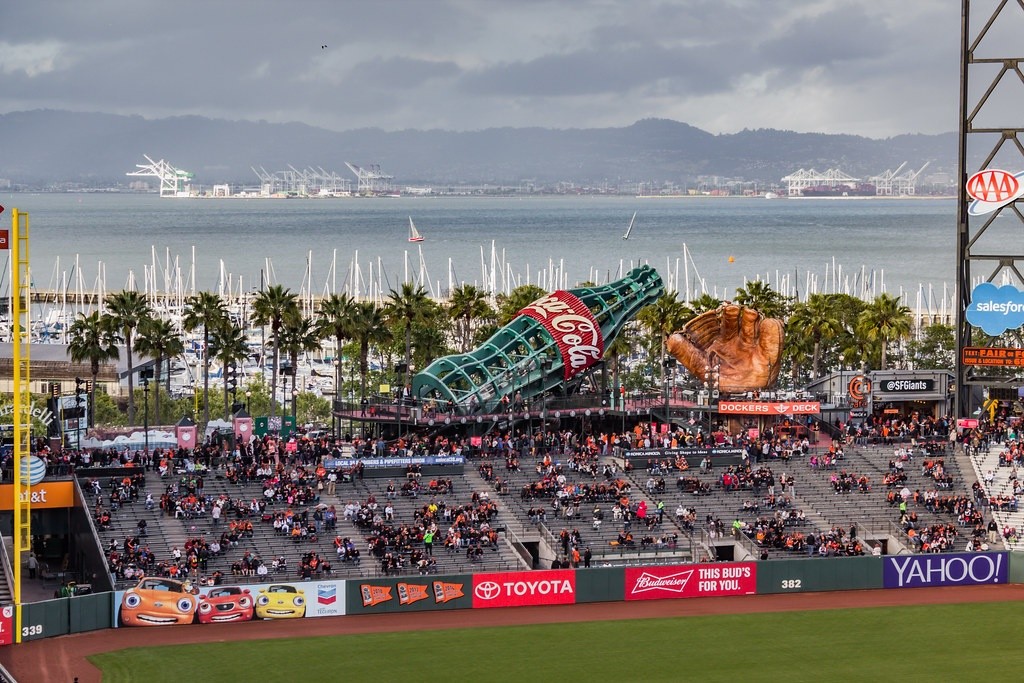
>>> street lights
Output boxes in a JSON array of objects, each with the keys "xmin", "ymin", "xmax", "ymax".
[
  {"xmin": 245, "ymin": 389, "xmax": 252, "ymax": 415},
  {"xmin": 856, "ymin": 359, "xmax": 871, "ymax": 430},
  {"xmin": 662, "ymin": 355, "xmax": 677, "ymax": 430},
  {"xmin": 394, "ymin": 363, "xmax": 408, "ymax": 436},
  {"xmin": 292, "ymin": 388, "xmax": 300, "ymax": 420},
  {"xmin": 333, "ymin": 357, "xmax": 341, "ymax": 401},
  {"xmin": 704, "ymin": 354, "xmax": 721, "ymax": 436},
  {"xmin": 839, "ymin": 353, "xmax": 845, "ymax": 402},
  {"xmin": 140, "ymin": 368, "xmax": 153, "ymax": 447},
  {"xmin": 279, "ymin": 362, "xmax": 294, "ymax": 447}
]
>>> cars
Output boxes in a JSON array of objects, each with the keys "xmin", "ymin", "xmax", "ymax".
[
  {"xmin": 196, "ymin": 586, "xmax": 255, "ymax": 624},
  {"xmin": 119, "ymin": 575, "xmax": 200, "ymax": 627},
  {"xmin": 255, "ymin": 585, "xmax": 307, "ymax": 621}
]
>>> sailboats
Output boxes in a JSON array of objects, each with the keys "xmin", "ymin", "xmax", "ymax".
[
  {"xmin": 621, "ymin": 211, "xmax": 636, "ymax": 239},
  {"xmin": 407, "ymin": 216, "xmax": 425, "ymax": 242},
  {"xmin": 0, "ymin": 232, "xmax": 1018, "ymax": 406}
]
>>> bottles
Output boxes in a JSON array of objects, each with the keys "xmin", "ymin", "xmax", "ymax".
[{"xmin": 412, "ymin": 264, "xmax": 664, "ymax": 418}]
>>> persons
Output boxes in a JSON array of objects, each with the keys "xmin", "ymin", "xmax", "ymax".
[{"xmin": 0, "ymin": 385, "xmax": 1024, "ymax": 600}]
